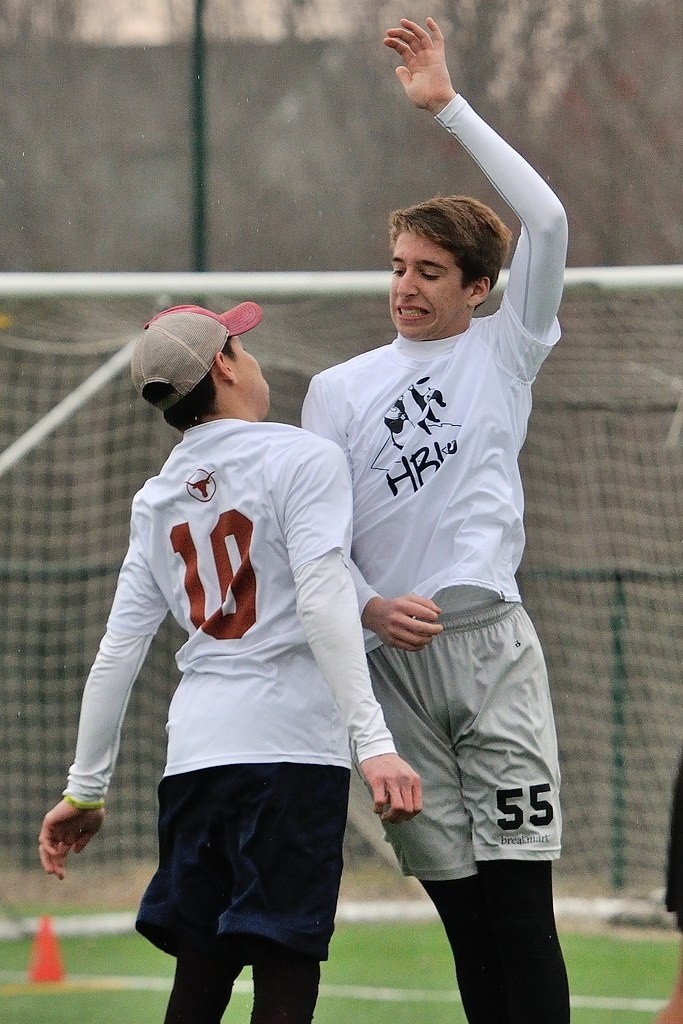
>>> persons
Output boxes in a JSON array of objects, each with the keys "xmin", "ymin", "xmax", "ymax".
[
  {"xmin": 654, "ymin": 757, "xmax": 683, "ymax": 1024},
  {"xmin": 38, "ymin": 301, "xmax": 421, "ymax": 1024},
  {"xmin": 302, "ymin": 18, "xmax": 574, "ymax": 1024}
]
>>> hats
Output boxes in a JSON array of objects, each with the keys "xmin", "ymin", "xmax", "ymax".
[{"xmin": 131, "ymin": 301, "xmax": 263, "ymax": 411}]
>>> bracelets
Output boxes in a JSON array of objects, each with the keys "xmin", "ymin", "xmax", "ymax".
[{"xmin": 64, "ymin": 795, "xmax": 105, "ymax": 809}]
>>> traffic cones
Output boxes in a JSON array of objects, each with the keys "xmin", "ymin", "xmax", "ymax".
[{"xmin": 29, "ymin": 915, "xmax": 61, "ymax": 983}]
